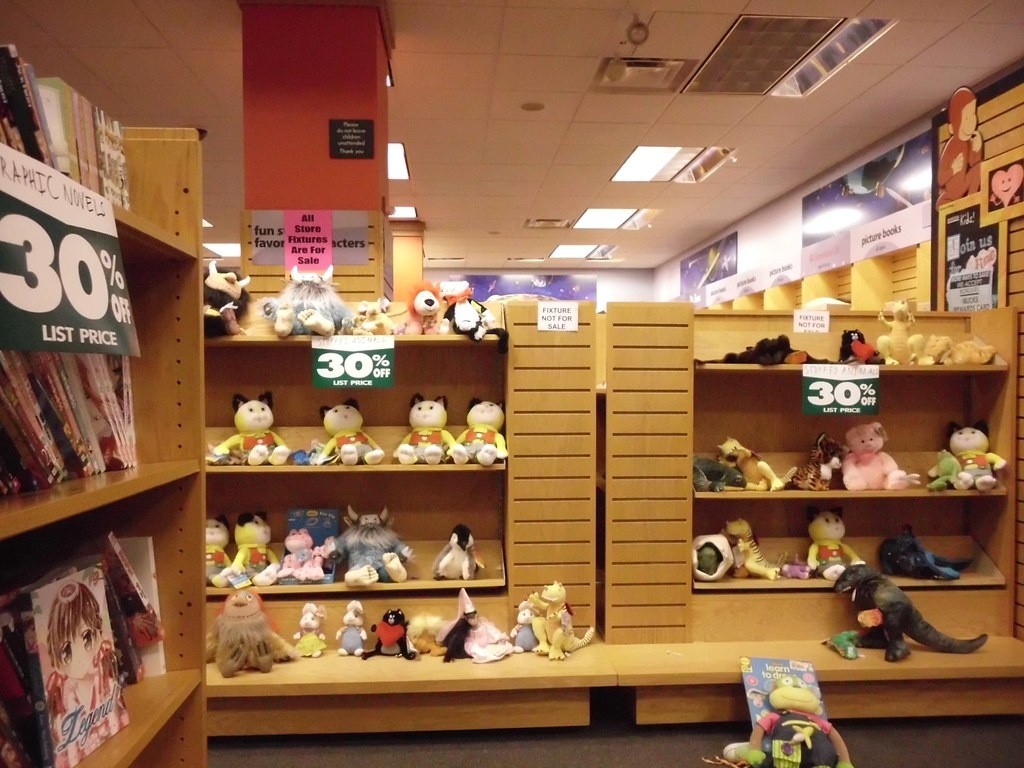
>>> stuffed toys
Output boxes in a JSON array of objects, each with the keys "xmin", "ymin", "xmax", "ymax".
[
  {"xmin": 199, "ymin": 256, "xmax": 496, "ymax": 345},
  {"xmin": 195, "ymin": 497, "xmax": 598, "ymax": 678},
  {"xmin": 692, "ymin": 422, "xmax": 1010, "ymax": 502},
  {"xmin": 208, "ymin": 392, "xmax": 509, "ymax": 467},
  {"xmin": 724, "ymin": 680, "xmax": 854, "ymax": 768},
  {"xmin": 693, "ymin": 300, "xmax": 1001, "ymax": 372},
  {"xmin": 692, "ymin": 512, "xmax": 993, "ymax": 664}
]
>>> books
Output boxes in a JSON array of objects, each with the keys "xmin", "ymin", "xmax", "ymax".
[
  {"xmin": 1, "ymin": 44, "xmax": 129, "ymax": 211},
  {"xmin": 0, "ymin": 528, "xmax": 168, "ymax": 768},
  {"xmin": 0, "ymin": 343, "xmax": 139, "ymax": 492},
  {"xmin": 739, "ymin": 655, "xmax": 830, "ymax": 736}
]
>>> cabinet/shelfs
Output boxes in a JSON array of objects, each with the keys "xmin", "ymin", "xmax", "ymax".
[{"xmin": 0, "ymin": 128, "xmax": 1024, "ymax": 768}]
